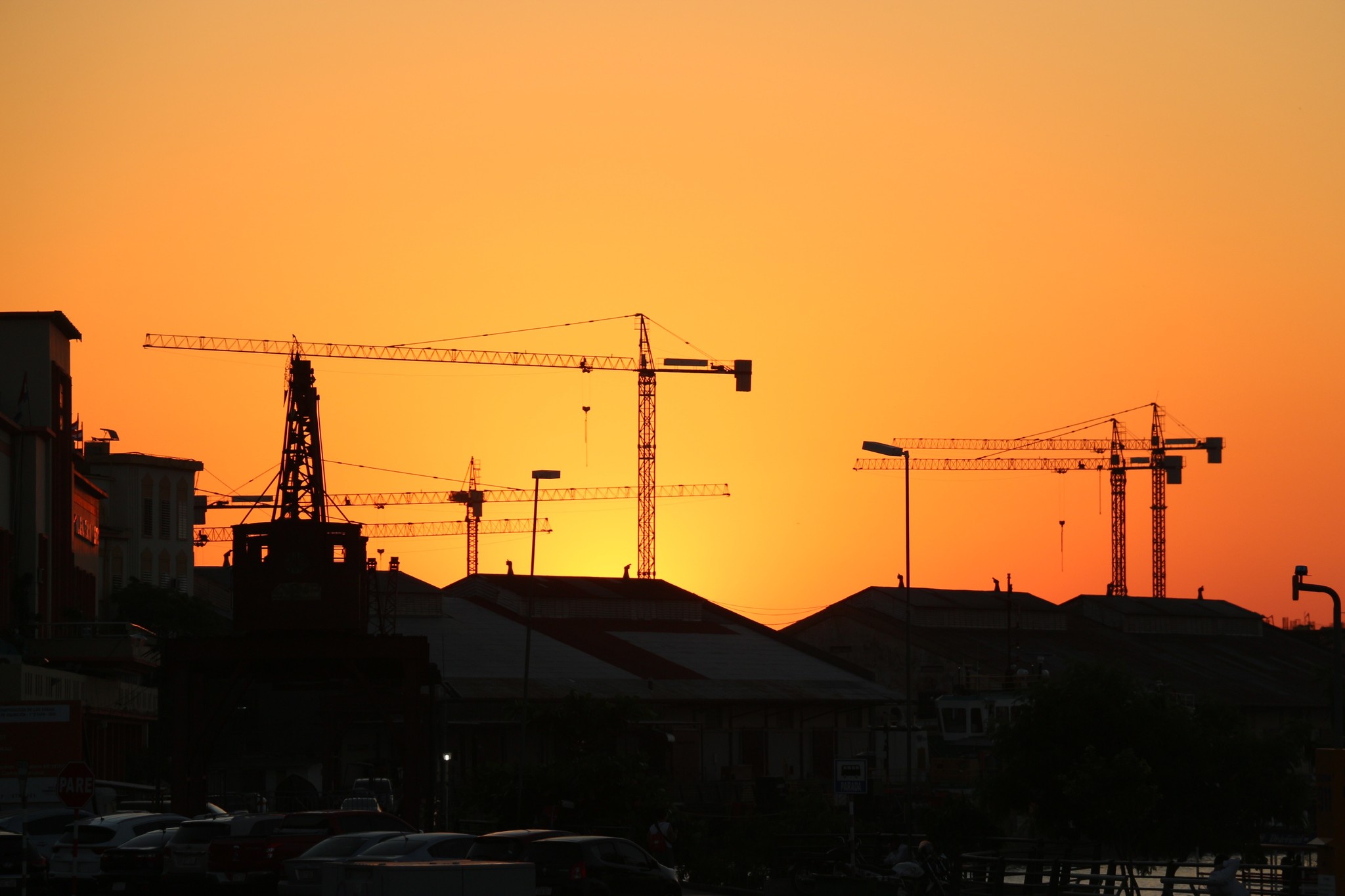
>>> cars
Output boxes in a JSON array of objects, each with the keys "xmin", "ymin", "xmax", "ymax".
[{"xmin": 2, "ymin": 776, "xmax": 686, "ymax": 896}]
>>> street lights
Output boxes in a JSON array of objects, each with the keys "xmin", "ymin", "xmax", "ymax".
[
  {"xmin": 862, "ymin": 440, "xmax": 914, "ymax": 834},
  {"xmin": 512, "ymin": 468, "xmax": 562, "ymax": 828}
]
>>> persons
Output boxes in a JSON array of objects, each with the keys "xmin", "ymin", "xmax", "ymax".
[
  {"xmin": 875, "ymin": 836, "xmax": 917, "ymax": 868},
  {"xmin": 1208, "ymin": 854, "xmax": 1252, "ymax": 896},
  {"xmin": 648, "ymin": 809, "xmax": 679, "ymax": 868},
  {"xmin": 1280, "ymin": 851, "xmax": 1306, "ymax": 896}
]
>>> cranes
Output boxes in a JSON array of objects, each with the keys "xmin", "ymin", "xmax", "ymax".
[
  {"xmin": 193, "ymin": 456, "xmax": 732, "ymax": 580},
  {"xmin": 849, "ymin": 401, "xmax": 1226, "ymax": 599},
  {"xmin": 143, "ymin": 311, "xmax": 756, "ymax": 581}
]
